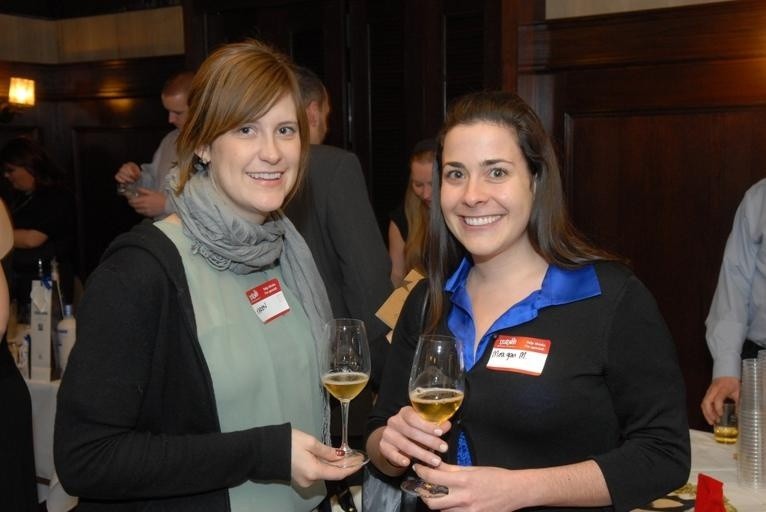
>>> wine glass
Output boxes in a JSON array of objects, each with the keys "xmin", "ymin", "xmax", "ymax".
[
  {"xmin": 319, "ymin": 316, "xmax": 372, "ymax": 467},
  {"xmin": 400, "ymin": 332, "xmax": 467, "ymax": 498}
]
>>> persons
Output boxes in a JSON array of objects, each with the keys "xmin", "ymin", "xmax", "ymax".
[
  {"xmin": 54, "ymin": 38, "xmax": 362, "ymax": 512},
  {"xmin": 3, "ymin": 131, "xmax": 97, "ymax": 323},
  {"xmin": 699, "ymin": 173, "xmax": 766, "ymax": 425},
  {"xmin": 114, "ymin": 70, "xmax": 208, "ymax": 222},
  {"xmin": 280, "ymin": 69, "xmax": 395, "ymax": 351},
  {"xmin": 362, "ymin": 90, "xmax": 691, "ymax": 512},
  {"xmin": 0, "ymin": 197, "xmax": 42, "ymax": 511},
  {"xmin": 388, "ymin": 139, "xmax": 437, "ymax": 288}
]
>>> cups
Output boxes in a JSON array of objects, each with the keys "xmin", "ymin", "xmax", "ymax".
[
  {"xmin": 712, "ymin": 403, "xmax": 738, "ymax": 444},
  {"xmin": 737, "ymin": 348, "xmax": 765, "ymax": 492}
]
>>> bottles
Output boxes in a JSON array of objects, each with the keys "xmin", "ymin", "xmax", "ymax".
[{"xmin": 54, "ymin": 305, "xmax": 82, "ymax": 377}]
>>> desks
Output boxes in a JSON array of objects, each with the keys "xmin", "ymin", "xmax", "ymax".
[{"xmin": 25, "ymin": 376, "xmax": 61, "ymax": 505}]
